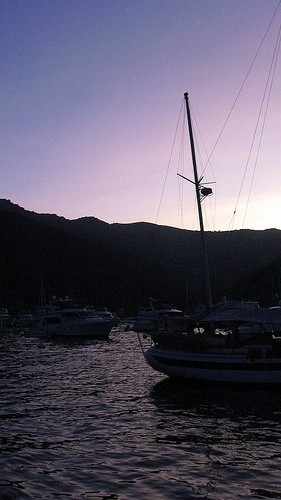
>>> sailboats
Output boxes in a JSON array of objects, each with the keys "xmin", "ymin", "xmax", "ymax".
[{"xmin": 139, "ymin": 91, "xmax": 281, "ymax": 383}]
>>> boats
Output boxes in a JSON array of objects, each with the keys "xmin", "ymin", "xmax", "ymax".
[
  {"xmin": 1, "ymin": 307, "xmax": 35, "ymax": 327},
  {"xmin": 27, "ymin": 295, "xmax": 113, "ymax": 338},
  {"xmin": 128, "ymin": 297, "xmax": 194, "ymax": 333}
]
